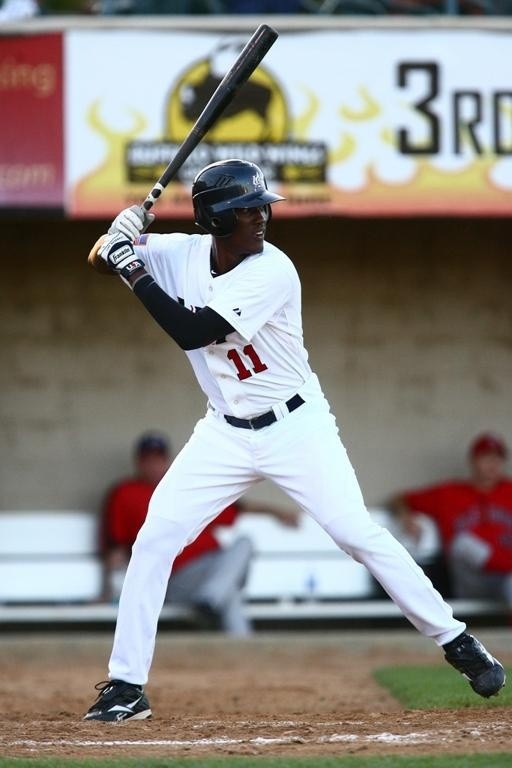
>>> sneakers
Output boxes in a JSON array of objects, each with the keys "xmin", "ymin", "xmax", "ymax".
[
  {"xmin": 444, "ymin": 634, "xmax": 507, "ymax": 698},
  {"xmin": 85, "ymin": 679, "xmax": 152, "ymax": 722}
]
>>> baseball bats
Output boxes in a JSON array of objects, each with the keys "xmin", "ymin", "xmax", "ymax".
[{"xmin": 143, "ymin": 25, "xmax": 280, "ymax": 210}]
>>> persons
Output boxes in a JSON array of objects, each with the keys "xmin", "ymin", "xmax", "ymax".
[
  {"xmin": 389, "ymin": 429, "xmax": 510, "ymax": 629},
  {"xmin": 82, "ymin": 159, "xmax": 506, "ymax": 727},
  {"xmin": 101, "ymin": 429, "xmax": 257, "ymax": 639}
]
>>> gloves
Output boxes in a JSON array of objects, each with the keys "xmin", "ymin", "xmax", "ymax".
[
  {"xmin": 108, "ymin": 204, "xmax": 155, "ymax": 242},
  {"xmin": 94, "ymin": 230, "xmax": 145, "ymax": 281}
]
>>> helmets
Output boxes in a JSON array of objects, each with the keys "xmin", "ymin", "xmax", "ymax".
[{"xmin": 191, "ymin": 159, "xmax": 287, "ymax": 239}]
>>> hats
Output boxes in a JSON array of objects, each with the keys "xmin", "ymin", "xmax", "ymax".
[
  {"xmin": 471, "ymin": 436, "xmax": 505, "ymax": 458},
  {"xmin": 134, "ymin": 436, "xmax": 167, "ymax": 454}
]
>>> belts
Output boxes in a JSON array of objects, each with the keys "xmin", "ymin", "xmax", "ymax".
[{"xmin": 225, "ymin": 394, "xmax": 304, "ymax": 430}]
